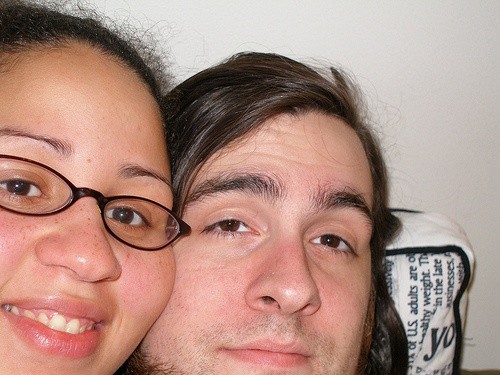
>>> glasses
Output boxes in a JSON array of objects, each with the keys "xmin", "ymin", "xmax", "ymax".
[{"xmin": 0, "ymin": 153, "xmax": 192, "ymax": 250}]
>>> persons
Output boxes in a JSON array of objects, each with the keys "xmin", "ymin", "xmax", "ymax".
[
  {"xmin": 0, "ymin": 0, "xmax": 175, "ymax": 374},
  {"xmin": 111, "ymin": 49, "xmax": 387, "ymax": 375}
]
